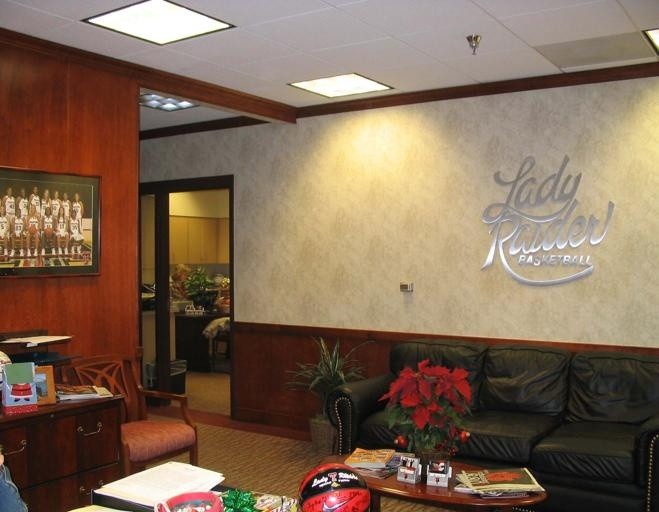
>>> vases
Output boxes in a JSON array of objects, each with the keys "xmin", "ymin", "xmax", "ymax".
[{"xmin": 410, "ymin": 443, "xmax": 452, "ymax": 483}]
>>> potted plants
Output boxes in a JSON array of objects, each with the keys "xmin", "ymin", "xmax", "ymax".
[
  {"xmin": 179, "ymin": 262, "xmax": 220, "ymax": 312},
  {"xmin": 280, "ymin": 330, "xmax": 380, "ymax": 458}
]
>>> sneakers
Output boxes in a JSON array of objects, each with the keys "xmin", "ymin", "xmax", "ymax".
[{"xmin": 0, "ymin": 245, "xmax": 81, "ymax": 257}]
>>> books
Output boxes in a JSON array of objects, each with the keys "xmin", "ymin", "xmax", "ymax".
[
  {"xmin": 94, "ymin": 461, "xmax": 225, "ymax": 509},
  {"xmin": 454, "ymin": 466, "xmax": 546, "ymax": 500},
  {"xmin": 3, "ymin": 361, "xmax": 114, "ymax": 405},
  {"xmin": 344, "ymin": 447, "xmax": 416, "ymax": 480}
]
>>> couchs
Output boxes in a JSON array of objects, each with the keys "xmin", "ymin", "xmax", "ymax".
[{"xmin": 324, "ymin": 334, "xmax": 659, "ymax": 512}]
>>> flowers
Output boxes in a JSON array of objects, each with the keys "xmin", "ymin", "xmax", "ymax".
[{"xmin": 375, "ymin": 354, "xmax": 477, "ymax": 460}]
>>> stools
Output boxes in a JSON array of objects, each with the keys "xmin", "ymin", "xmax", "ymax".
[{"xmin": 202, "ymin": 315, "xmax": 231, "ymax": 373}]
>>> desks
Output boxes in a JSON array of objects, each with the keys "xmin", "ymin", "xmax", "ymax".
[
  {"xmin": 141, "ymin": 304, "xmax": 181, "ymax": 364},
  {"xmin": 172, "ymin": 309, "xmax": 231, "ymax": 372},
  {"xmin": 76, "ymin": 481, "xmax": 303, "ymax": 511}
]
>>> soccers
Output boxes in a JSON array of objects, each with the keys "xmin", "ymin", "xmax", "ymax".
[{"xmin": 298, "ymin": 463, "xmax": 371, "ymax": 512}]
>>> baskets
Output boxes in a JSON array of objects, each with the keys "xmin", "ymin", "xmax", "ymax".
[
  {"xmin": 216, "ymin": 304, "xmax": 231, "ymax": 315},
  {"xmin": 170, "ymin": 300, "xmax": 205, "ymax": 316}
]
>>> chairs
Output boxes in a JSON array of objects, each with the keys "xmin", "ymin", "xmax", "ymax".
[{"xmin": 57, "ymin": 352, "xmax": 199, "ymax": 479}]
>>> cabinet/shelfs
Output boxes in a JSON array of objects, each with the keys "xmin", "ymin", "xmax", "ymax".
[
  {"xmin": 167, "ymin": 214, "xmax": 187, "ymax": 266},
  {"xmin": 187, "ymin": 216, "xmax": 218, "ymax": 264},
  {"xmin": 217, "ymin": 217, "xmax": 230, "ymax": 265},
  {"xmin": 1, "ymin": 382, "xmax": 126, "ymax": 511}
]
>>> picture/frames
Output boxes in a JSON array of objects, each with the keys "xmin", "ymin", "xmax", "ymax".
[
  {"xmin": 0, "ymin": 164, "xmax": 105, "ymax": 281},
  {"xmin": 32, "ymin": 365, "xmax": 59, "ymax": 406}
]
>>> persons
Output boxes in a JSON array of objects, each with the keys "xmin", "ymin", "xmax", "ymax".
[
  {"xmin": 0, "ymin": 453, "xmax": 28, "ymax": 512},
  {"xmin": 0, "ymin": 186, "xmax": 84, "ymax": 258},
  {"xmin": 169, "ymin": 263, "xmax": 192, "ymax": 300}
]
